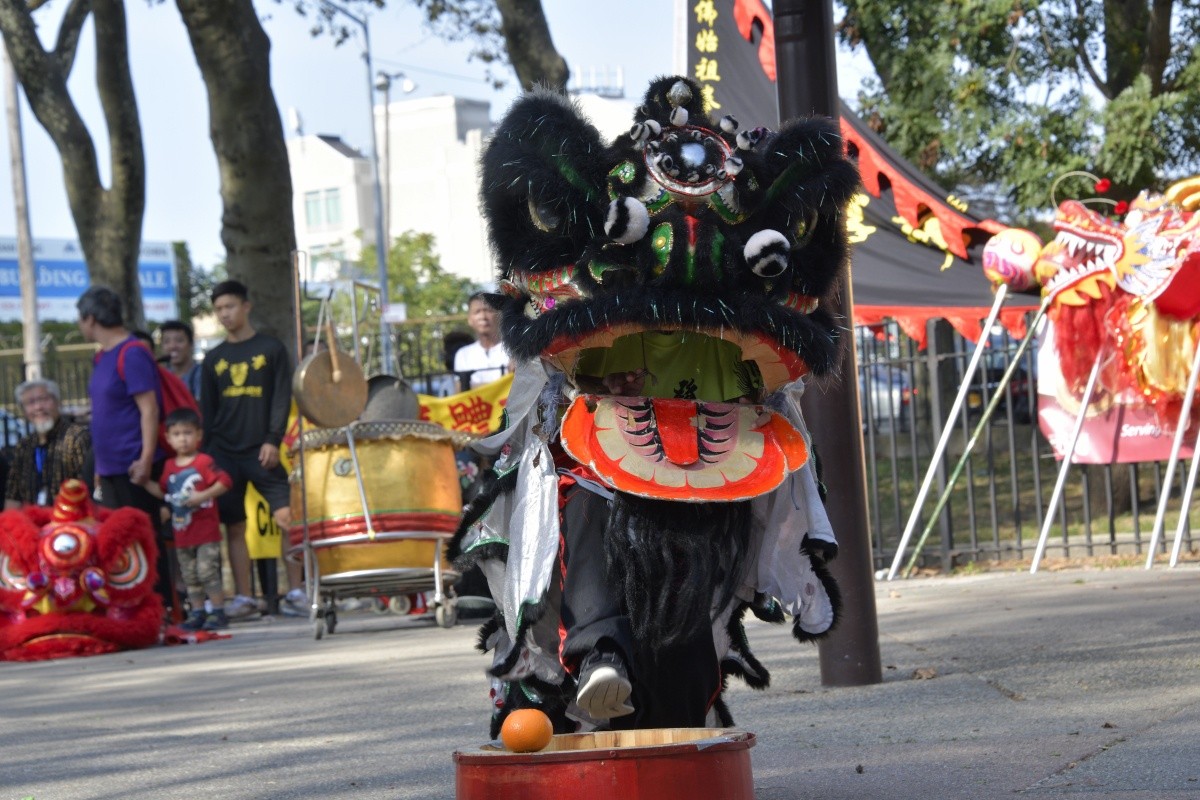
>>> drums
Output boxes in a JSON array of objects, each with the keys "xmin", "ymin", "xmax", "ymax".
[{"xmin": 286, "ymin": 419, "xmax": 465, "ymax": 582}]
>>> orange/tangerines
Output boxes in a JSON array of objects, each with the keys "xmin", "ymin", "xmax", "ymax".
[{"xmin": 502, "ymin": 709, "xmax": 553, "ymax": 753}]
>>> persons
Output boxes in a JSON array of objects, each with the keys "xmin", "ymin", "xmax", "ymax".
[
  {"xmin": 77, "ymin": 285, "xmax": 227, "ymax": 631},
  {"xmin": 4, "ymin": 379, "xmax": 95, "ymax": 516},
  {"xmin": 454, "ymin": 293, "xmax": 514, "ymax": 394},
  {"xmin": 202, "ymin": 280, "xmax": 314, "ymax": 623}
]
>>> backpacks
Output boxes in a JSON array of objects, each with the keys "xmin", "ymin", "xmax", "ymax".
[{"xmin": 95, "ymin": 340, "xmax": 203, "ymax": 455}]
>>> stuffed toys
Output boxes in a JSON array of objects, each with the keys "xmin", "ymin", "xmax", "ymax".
[
  {"xmin": 0, "ymin": 480, "xmax": 163, "ymax": 662},
  {"xmin": 445, "ymin": 71, "xmax": 858, "ymax": 736}
]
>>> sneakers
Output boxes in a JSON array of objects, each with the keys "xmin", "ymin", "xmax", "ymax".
[
  {"xmin": 223, "ymin": 593, "xmax": 262, "ymax": 621},
  {"xmin": 205, "ymin": 600, "xmax": 213, "ymax": 614},
  {"xmin": 576, "ymin": 651, "xmax": 635, "ymax": 721},
  {"xmin": 202, "ymin": 608, "xmax": 229, "ymax": 629},
  {"xmin": 179, "ymin": 609, "xmax": 207, "ymax": 630},
  {"xmin": 283, "ymin": 593, "xmax": 325, "ymax": 617}
]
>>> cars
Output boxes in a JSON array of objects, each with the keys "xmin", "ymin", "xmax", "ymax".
[{"xmin": 856, "ymin": 318, "xmax": 1042, "ymax": 434}]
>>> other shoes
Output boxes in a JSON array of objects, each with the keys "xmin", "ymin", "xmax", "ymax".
[{"xmin": 157, "ymin": 613, "xmax": 174, "ymax": 641}]
